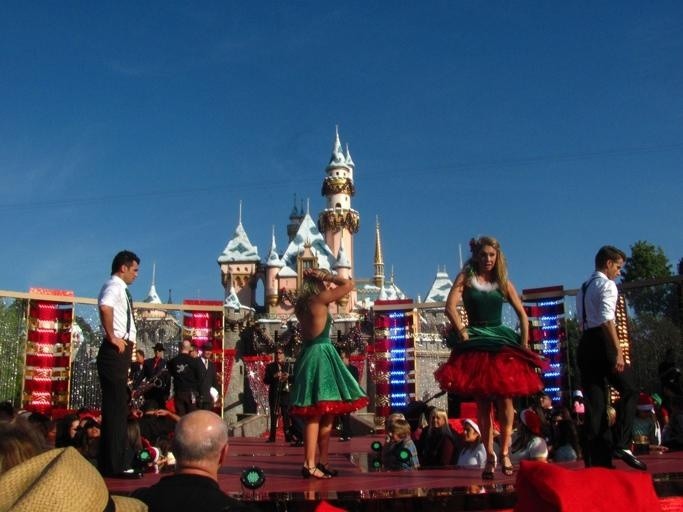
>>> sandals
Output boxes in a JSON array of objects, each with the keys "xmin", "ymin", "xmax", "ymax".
[
  {"xmin": 497, "ymin": 449, "xmax": 514, "ymax": 476},
  {"xmin": 481, "ymin": 453, "xmax": 499, "ymax": 480}
]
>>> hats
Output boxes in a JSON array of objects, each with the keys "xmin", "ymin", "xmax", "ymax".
[
  {"xmin": 151, "ymin": 342, "xmax": 167, "ymax": 353},
  {"xmin": 518, "ymin": 409, "xmax": 542, "ymax": 433},
  {"xmin": 637, "ymin": 391, "xmax": 654, "ymax": 411},
  {"xmin": 199, "ymin": 343, "xmax": 215, "ymax": 351},
  {"xmin": 0, "ymin": 444, "xmax": 149, "ymax": 512},
  {"xmin": 78, "ymin": 409, "xmax": 101, "ymax": 428},
  {"xmin": 463, "ymin": 417, "xmax": 481, "ymax": 437}
]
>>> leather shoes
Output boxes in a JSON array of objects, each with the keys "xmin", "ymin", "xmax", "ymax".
[
  {"xmin": 119, "ymin": 467, "xmax": 144, "ymax": 480},
  {"xmin": 611, "ymin": 448, "xmax": 648, "ymax": 471}
]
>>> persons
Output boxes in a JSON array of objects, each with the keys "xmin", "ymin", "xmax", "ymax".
[
  {"xmin": 575, "ymin": 245, "xmax": 647, "ymax": 472},
  {"xmin": 1, "ymin": 340, "xmax": 252, "ymax": 512},
  {"xmin": 263, "ymin": 347, "xmax": 292, "ymax": 443},
  {"xmin": 432, "ymin": 235, "xmax": 551, "ymax": 481},
  {"xmin": 96, "ymin": 249, "xmax": 142, "ymax": 480},
  {"xmin": 380, "ymin": 389, "xmax": 669, "ymax": 474},
  {"xmin": 286, "ymin": 266, "xmax": 370, "ymax": 480}
]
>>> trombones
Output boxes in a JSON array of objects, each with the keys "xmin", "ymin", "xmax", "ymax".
[{"xmin": 274, "ymin": 359, "xmax": 291, "ymax": 416}]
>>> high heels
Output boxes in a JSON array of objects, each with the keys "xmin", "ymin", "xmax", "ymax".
[
  {"xmin": 316, "ymin": 461, "xmax": 339, "ymax": 478},
  {"xmin": 300, "ymin": 465, "xmax": 332, "ymax": 480}
]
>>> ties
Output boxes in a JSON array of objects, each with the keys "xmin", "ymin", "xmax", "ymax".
[{"xmin": 205, "ymin": 360, "xmax": 208, "ymax": 370}]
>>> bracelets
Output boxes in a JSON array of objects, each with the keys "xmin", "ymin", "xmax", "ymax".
[
  {"xmin": 456, "ymin": 325, "xmax": 466, "ymax": 337},
  {"xmin": 319, "ymin": 272, "xmax": 328, "ymax": 281}
]
